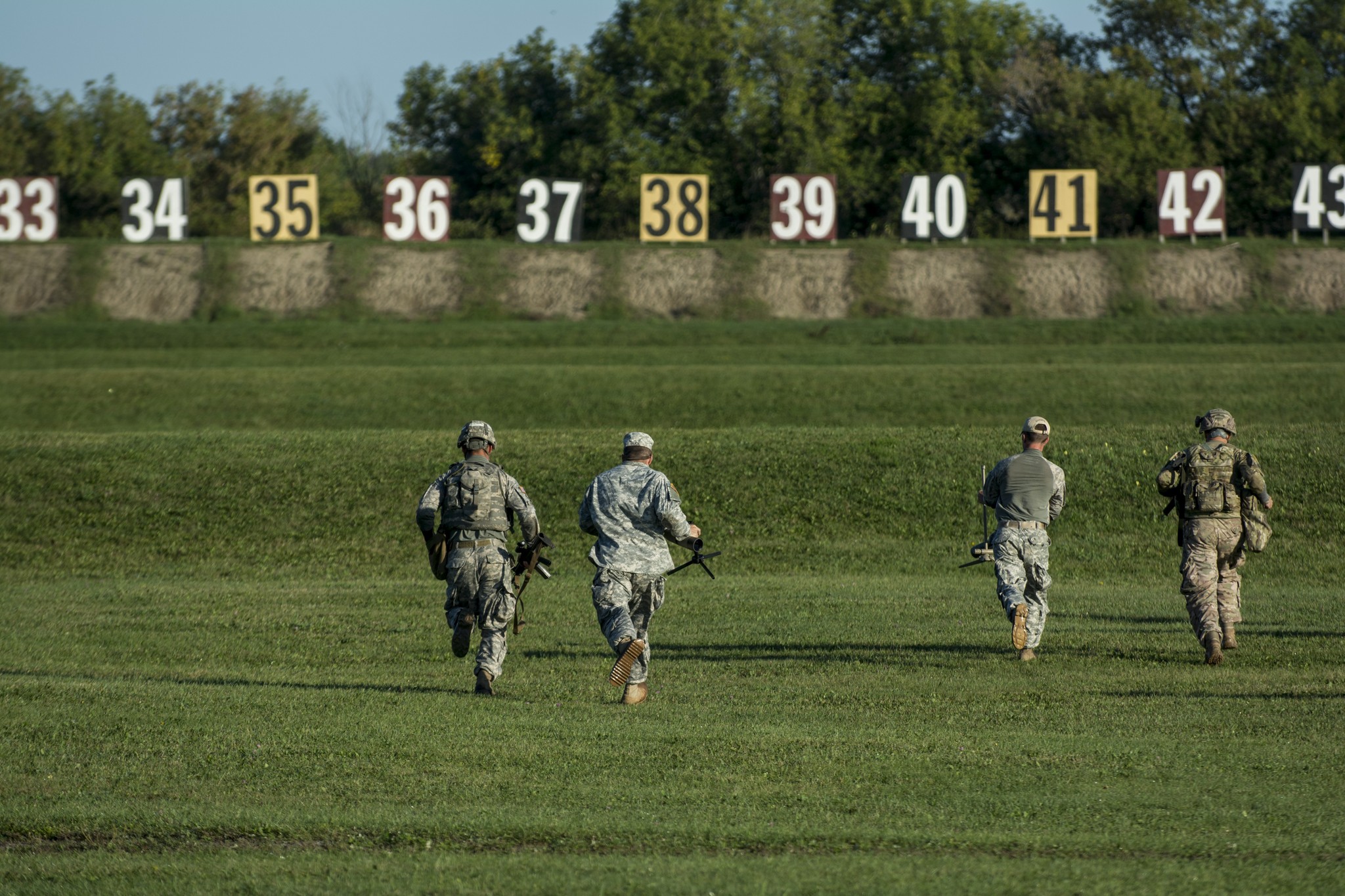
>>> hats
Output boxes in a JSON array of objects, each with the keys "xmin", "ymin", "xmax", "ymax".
[
  {"xmin": 1019, "ymin": 417, "xmax": 1050, "ymax": 436},
  {"xmin": 623, "ymin": 432, "xmax": 654, "ymax": 450}
]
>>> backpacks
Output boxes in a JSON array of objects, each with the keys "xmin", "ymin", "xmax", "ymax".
[{"xmin": 462, "ymin": 468, "xmax": 493, "ymax": 511}]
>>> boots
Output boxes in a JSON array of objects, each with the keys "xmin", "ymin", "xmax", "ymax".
[
  {"xmin": 1220, "ymin": 623, "xmax": 1237, "ymax": 650},
  {"xmin": 1202, "ymin": 632, "xmax": 1223, "ymax": 665}
]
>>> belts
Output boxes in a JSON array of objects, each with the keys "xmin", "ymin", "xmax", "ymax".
[
  {"xmin": 997, "ymin": 520, "xmax": 1046, "ymax": 530},
  {"xmin": 451, "ymin": 539, "xmax": 506, "ymax": 549}
]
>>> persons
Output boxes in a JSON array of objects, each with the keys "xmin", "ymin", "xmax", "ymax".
[
  {"xmin": 1156, "ymin": 410, "xmax": 1272, "ymax": 663},
  {"xmin": 984, "ymin": 415, "xmax": 1067, "ymax": 661},
  {"xmin": 415, "ymin": 420, "xmax": 542, "ymax": 697},
  {"xmin": 581, "ymin": 432, "xmax": 701, "ymax": 705}
]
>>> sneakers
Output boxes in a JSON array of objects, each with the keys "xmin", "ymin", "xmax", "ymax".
[
  {"xmin": 621, "ymin": 683, "xmax": 647, "ymax": 705},
  {"xmin": 476, "ymin": 669, "xmax": 496, "ymax": 696},
  {"xmin": 1011, "ymin": 603, "xmax": 1027, "ymax": 650},
  {"xmin": 610, "ymin": 637, "xmax": 645, "ymax": 688},
  {"xmin": 1020, "ymin": 648, "xmax": 1038, "ymax": 661},
  {"xmin": 451, "ymin": 609, "xmax": 476, "ymax": 659}
]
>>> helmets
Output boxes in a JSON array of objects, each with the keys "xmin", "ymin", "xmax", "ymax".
[
  {"xmin": 457, "ymin": 419, "xmax": 497, "ymax": 449},
  {"xmin": 1198, "ymin": 409, "xmax": 1237, "ymax": 436}
]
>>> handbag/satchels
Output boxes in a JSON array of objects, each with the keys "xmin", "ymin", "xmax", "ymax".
[
  {"xmin": 1181, "ymin": 480, "xmax": 1237, "ymax": 513},
  {"xmin": 427, "ymin": 533, "xmax": 447, "ymax": 580},
  {"xmin": 1242, "ymin": 507, "xmax": 1273, "ymax": 553}
]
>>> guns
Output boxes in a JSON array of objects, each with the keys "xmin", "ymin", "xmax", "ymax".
[
  {"xmin": 512, "ymin": 529, "xmax": 556, "ymax": 585},
  {"xmin": 1162, "ymin": 495, "xmax": 1184, "ymax": 551}
]
